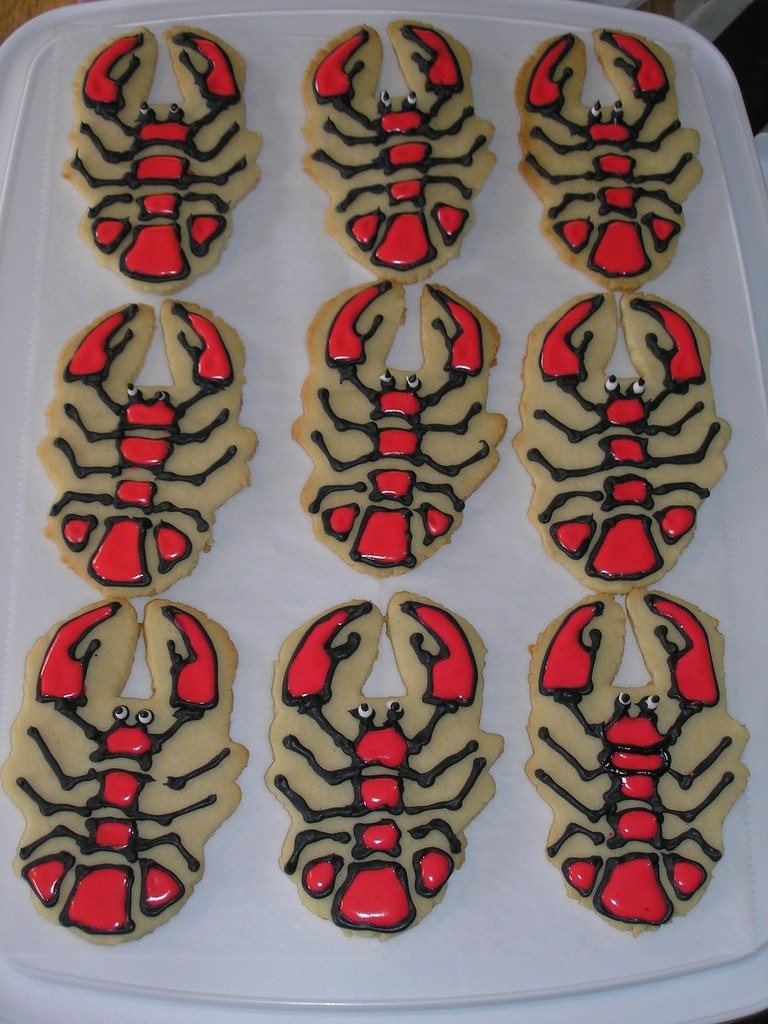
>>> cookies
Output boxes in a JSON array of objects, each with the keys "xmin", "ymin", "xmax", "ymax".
[{"xmin": 0, "ymin": 19, "xmax": 750, "ymax": 943}]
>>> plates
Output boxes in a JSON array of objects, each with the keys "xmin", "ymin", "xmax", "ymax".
[{"xmin": 0, "ymin": 0, "xmax": 768, "ymax": 1024}]
